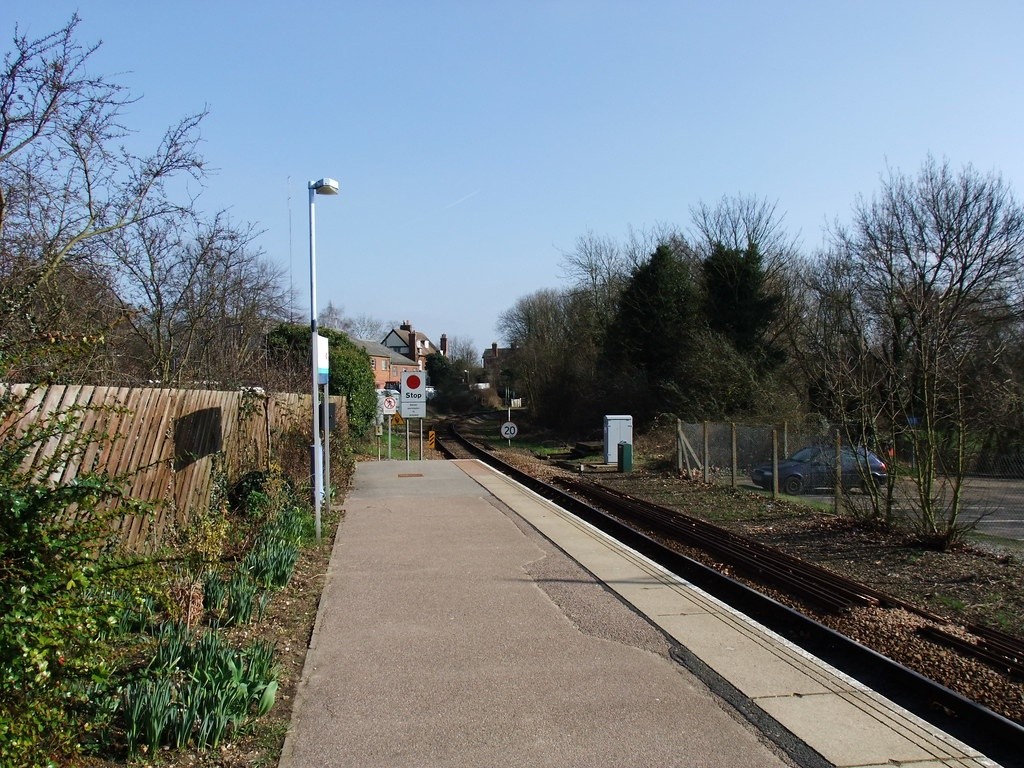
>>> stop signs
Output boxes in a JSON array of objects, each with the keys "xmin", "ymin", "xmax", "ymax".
[{"xmin": 400, "ymin": 371, "xmax": 427, "ymax": 403}]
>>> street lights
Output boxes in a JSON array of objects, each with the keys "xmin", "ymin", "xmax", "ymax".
[
  {"xmin": 465, "ymin": 369, "xmax": 470, "ymax": 384},
  {"xmin": 305, "ymin": 177, "xmax": 340, "ymax": 507}
]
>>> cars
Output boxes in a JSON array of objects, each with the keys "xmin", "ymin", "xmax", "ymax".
[{"xmin": 749, "ymin": 444, "xmax": 889, "ymax": 497}]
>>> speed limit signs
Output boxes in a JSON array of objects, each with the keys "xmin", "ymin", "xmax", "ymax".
[{"xmin": 500, "ymin": 421, "xmax": 518, "ymax": 439}]
